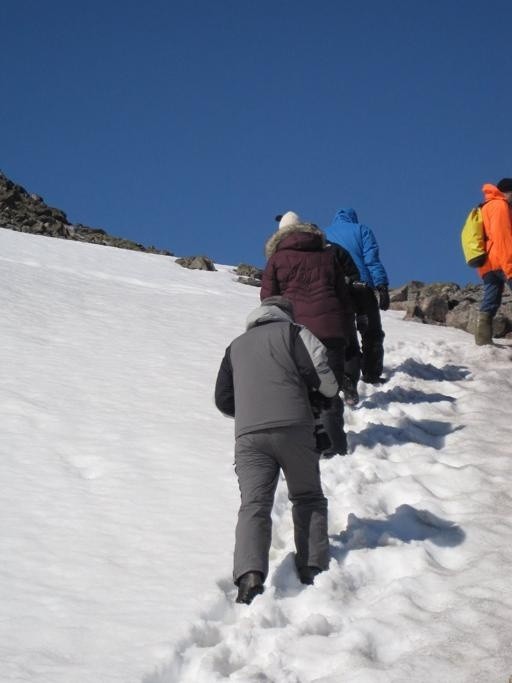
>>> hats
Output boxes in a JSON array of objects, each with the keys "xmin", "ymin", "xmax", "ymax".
[
  {"xmin": 261, "ymin": 296, "xmax": 293, "ymax": 319},
  {"xmin": 497, "ymin": 178, "xmax": 512, "ymax": 192},
  {"xmin": 276, "ymin": 210, "xmax": 303, "ymax": 228}
]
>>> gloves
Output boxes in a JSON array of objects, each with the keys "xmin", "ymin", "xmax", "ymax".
[{"xmin": 377, "ymin": 283, "xmax": 390, "ymax": 310}]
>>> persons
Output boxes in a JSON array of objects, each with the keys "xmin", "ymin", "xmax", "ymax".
[
  {"xmin": 259, "ymin": 211, "xmax": 362, "ymax": 457},
  {"xmin": 323, "ymin": 208, "xmax": 389, "ymax": 385},
  {"xmin": 461, "ymin": 179, "xmax": 512, "ymax": 348},
  {"xmin": 212, "ymin": 294, "xmax": 338, "ymax": 605}
]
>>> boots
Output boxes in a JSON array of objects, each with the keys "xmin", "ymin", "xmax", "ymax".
[{"xmin": 475, "ymin": 311, "xmax": 505, "ymax": 348}]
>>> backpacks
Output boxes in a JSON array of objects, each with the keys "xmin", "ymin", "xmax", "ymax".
[{"xmin": 461, "ymin": 200, "xmax": 487, "ymax": 267}]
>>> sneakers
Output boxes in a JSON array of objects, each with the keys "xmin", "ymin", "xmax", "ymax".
[
  {"xmin": 235, "ymin": 572, "xmax": 263, "ymax": 604},
  {"xmin": 342, "ymin": 377, "xmax": 359, "ymax": 405}
]
